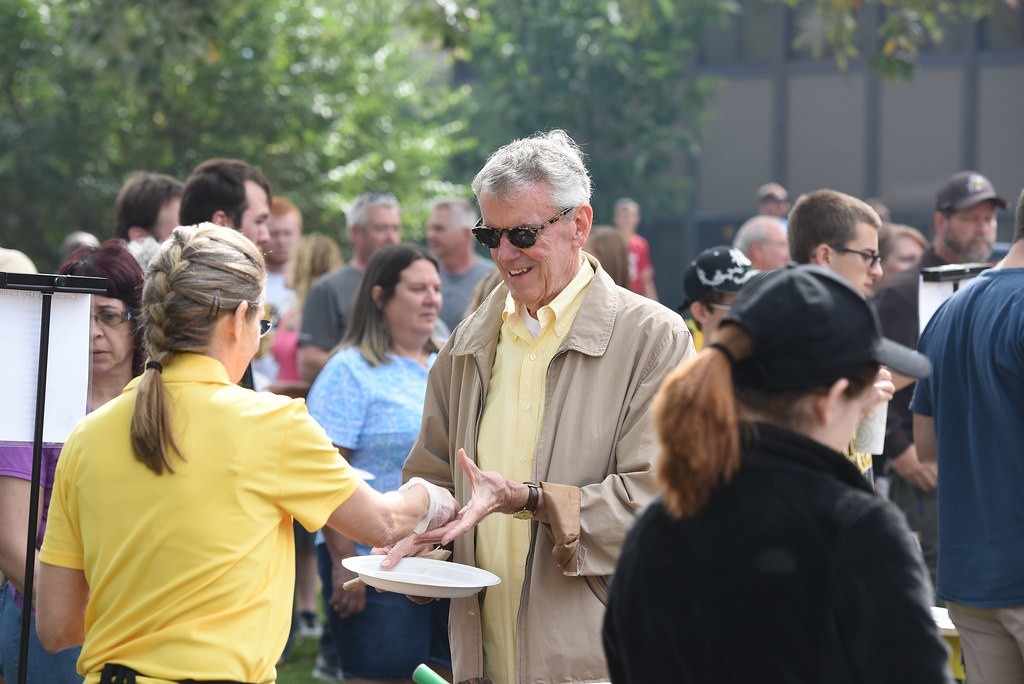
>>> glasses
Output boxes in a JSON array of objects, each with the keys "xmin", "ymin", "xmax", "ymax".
[
  {"xmin": 91, "ymin": 311, "xmax": 134, "ymax": 328},
  {"xmin": 470, "ymin": 207, "xmax": 573, "ymax": 250},
  {"xmin": 233, "ymin": 299, "xmax": 272, "ymax": 337},
  {"xmin": 811, "ymin": 243, "xmax": 883, "ymax": 269}
]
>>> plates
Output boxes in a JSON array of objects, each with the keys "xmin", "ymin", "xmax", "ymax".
[{"xmin": 342, "ymin": 555, "xmax": 500, "ymax": 597}]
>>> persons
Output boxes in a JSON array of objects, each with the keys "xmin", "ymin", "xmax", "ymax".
[
  {"xmin": 674, "ymin": 173, "xmax": 1024, "ymax": 684},
  {"xmin": 604, "ymin": 265, "xmax": 954, "ymax": 684},
  {"xmin": 0, "ymin": 159, "xmax": 658, "ymax": 684},
  {"xmin": 32, "ymin": 220, "xmax": 461, "ymax": 684},
  {"xmin": 371, "ymin": 131, "xmax": 697, "ymax": 684}
]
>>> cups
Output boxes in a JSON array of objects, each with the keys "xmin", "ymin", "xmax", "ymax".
[{"xmin": 852, "ymin": 398, "xmax": 888, "ymax": 456}]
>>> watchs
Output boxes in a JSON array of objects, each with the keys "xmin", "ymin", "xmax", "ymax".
[{"xmin": 513, "ymin": 481, "xmax": 539, "ymax": 521}]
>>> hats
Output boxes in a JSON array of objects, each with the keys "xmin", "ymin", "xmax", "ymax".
[
  {"xmin": 716, "ymin": 263, "xmax": 931, "ymax": 391},
  {"xmin": 934, "ymin": 172, "xmax": 1009, "ymax": 210},
  {"xmin": 682, "ymin": 245, "xmax": 777, "ymax": 297}
]
